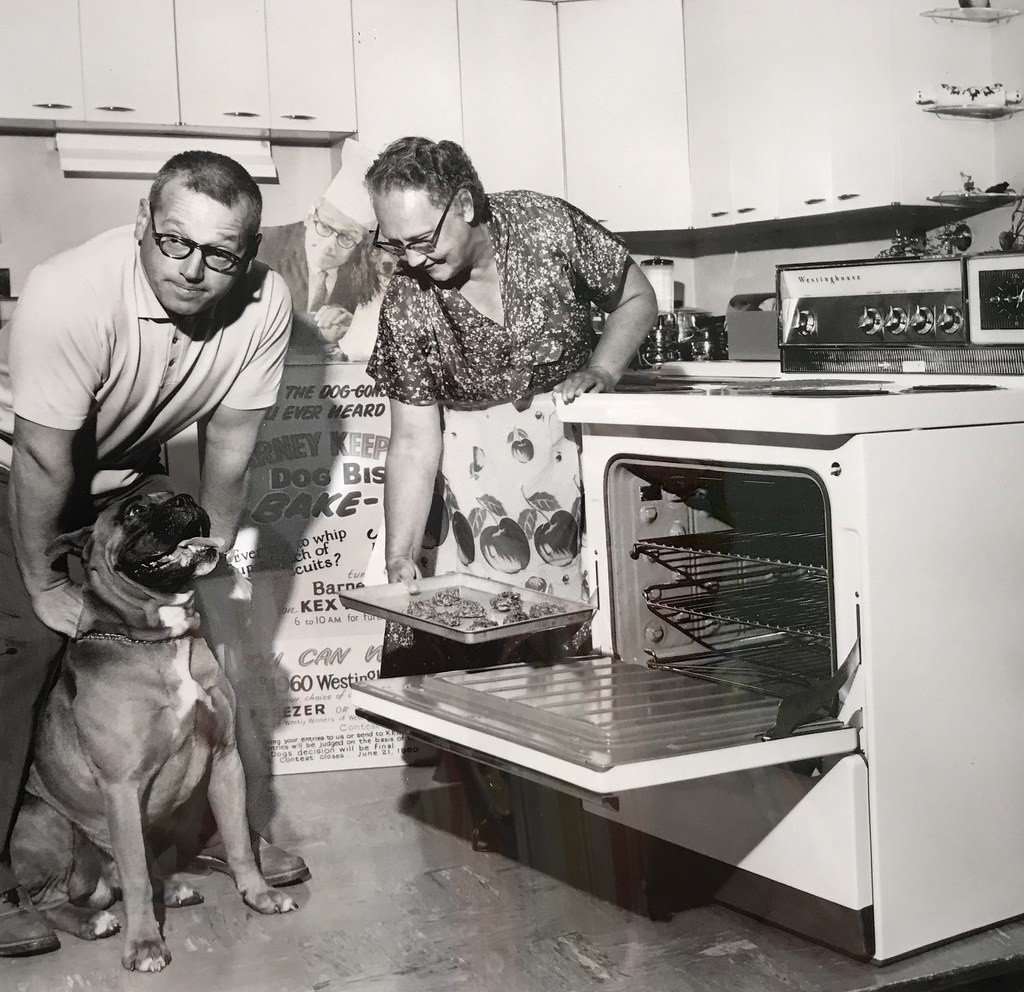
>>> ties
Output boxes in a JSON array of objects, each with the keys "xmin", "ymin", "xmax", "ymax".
[{"xmin": 310, "ymin": 271, "xmax": 330, "ymax": 312}]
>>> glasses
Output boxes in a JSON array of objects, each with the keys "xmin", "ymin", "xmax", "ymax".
[
  {"xmin": 148, "ymin": 209, "xmax": 253, "ymax": 274},
  {"xmin": 313, "ymin": 208, "xmax": 362, "ymax": 249},
  {"xmin": 371, "ymin": 190, "xmax": 458, "ymax": 255}
]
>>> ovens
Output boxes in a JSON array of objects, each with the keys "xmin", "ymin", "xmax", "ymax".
[{"xmin": 344, "ymin": 382, "xmax": 1024, "ymax": 967}]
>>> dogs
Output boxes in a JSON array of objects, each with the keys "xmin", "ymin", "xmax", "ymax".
[{"xmin": 7, "ymin": 484, "xmax": 301, "ymax": 973}]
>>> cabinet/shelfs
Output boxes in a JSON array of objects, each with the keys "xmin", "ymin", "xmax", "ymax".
[
  {"xmin": 920, "ymin": 7, "xmax": 1024, "ymax": 204},
  {"xmin": 322, "ymin": 0, "xmax": 569, "ymax": 195},
  {"xmin": 0, "ymin": 0, "xmax": 181, "ymax": 133},
  {"xmin": 682, "ymin": 0, "xmax": 995, "ymax": 242},
  {"xmin": 558, "ymin": 0, "xmax": 692, "ymax": 243},
  {"xmin": 174, "ymin": 0, "xmax": 358, "ymax": 144}
]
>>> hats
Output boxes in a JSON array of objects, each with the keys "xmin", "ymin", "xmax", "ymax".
[{"xmin": 323, "ymin": 135, "xmax": 389, "ymax": 234}]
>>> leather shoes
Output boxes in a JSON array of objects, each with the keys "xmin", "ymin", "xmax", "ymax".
[
  {"xmin": 175, "ymin": 824, "xmax": 309, "ymax": 887},
  {"xmin": 0, "ymin": 872, "xmax": 60, "ymax": 956}
]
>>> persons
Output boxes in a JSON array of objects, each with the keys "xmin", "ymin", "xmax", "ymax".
[
  {"xmin": 0, "ymin": 151, "xmax": 293, "ymax": 956},
  {"xmin": 368, "ymin": 139, "xmax": 660, "ymax": 681},
  {"xmin": 250, "ymin": 165, "xmax": 377, "ymax": 353}
]
